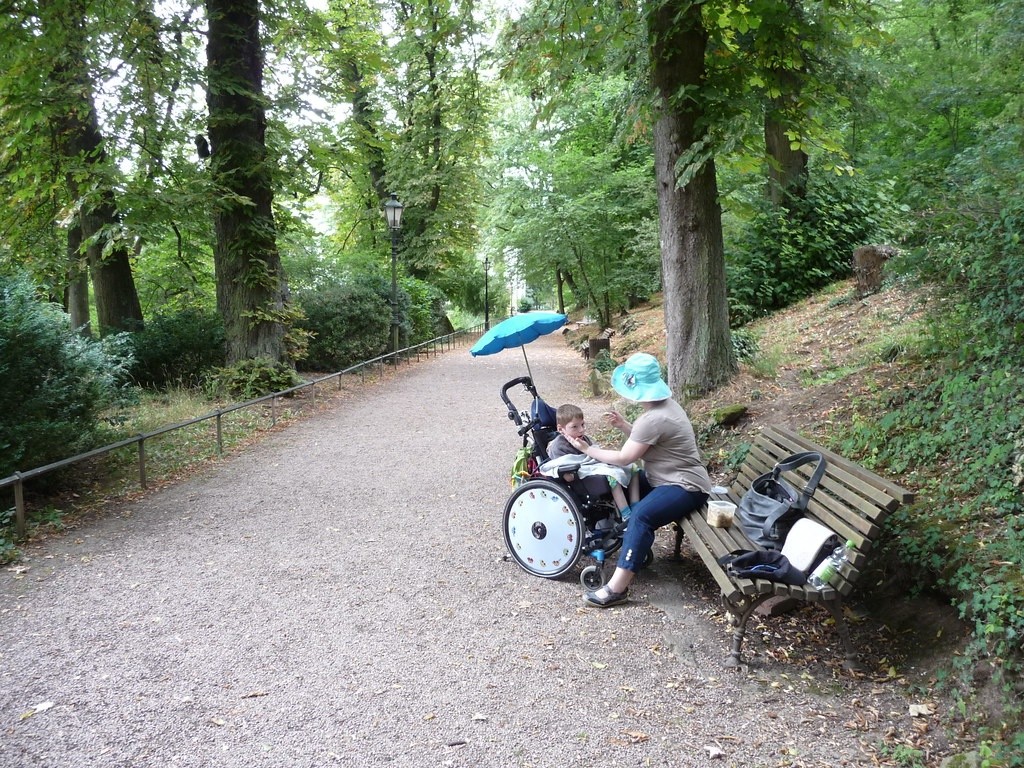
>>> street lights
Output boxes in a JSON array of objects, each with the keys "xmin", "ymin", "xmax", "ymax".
[
  {"xmin": 385, "ymin": 193, "xmax": 403, "ymax": 364},
  {"xmin": 483, "ymin": 256, "xmax": 491, "ymax": 331}
]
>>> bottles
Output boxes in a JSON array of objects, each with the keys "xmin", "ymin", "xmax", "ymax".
[{"xmin": 807, "ymin": 540, "xmax": 855, "ymax": 591}]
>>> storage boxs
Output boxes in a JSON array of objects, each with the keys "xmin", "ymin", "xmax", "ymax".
[{"xmin": 706, "ymin": 500, "xmax": 738, "ymax": 528}]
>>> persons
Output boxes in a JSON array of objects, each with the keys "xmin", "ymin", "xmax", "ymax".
[
  {"xmin": 548, "ymin": 404, "xmax": 641, "ymax": 522},
  {"xmin": 564, "ymin": 353, "xmax": 711, "ymax": 608}
]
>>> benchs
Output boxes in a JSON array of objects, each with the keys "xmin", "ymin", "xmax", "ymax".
[
  {"xmin": 580, "ymin": 327, "xmax": 615, "ymax": 360},
  {"xmin": 673, "ymin": 422, "xmax": 914, "ymax": 670}
]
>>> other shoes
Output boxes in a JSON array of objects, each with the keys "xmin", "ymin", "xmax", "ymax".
[{"xmin": 582, "ymin": 585, "xmax": 628, "ymax": 608}]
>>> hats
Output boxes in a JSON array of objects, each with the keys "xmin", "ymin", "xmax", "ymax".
[
  {"xmin": 610, "ymin": 352, "xmax": 673, "ymax": 403},
  {"xmin": 727, "ymin": 550, "xmax": 807, "ymax": 584}
]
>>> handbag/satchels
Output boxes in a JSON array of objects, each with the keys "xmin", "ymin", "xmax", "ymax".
[
  {"xmin": 738, "ymin": 450, "xmax": 828, "ymax": 549},
  {"xmin": 780, "ymin": 517, "xmax": 840, "ymax": 575}
]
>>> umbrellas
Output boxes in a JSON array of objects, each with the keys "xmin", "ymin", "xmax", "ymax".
[{"xmin": 469, "ymin": 312, "xmax": 568, "ymax": 385}]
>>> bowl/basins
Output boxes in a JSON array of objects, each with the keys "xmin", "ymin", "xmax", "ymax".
[{"xmin": 706, "ymin": 500, "xmax": 737, "ymax": 528}]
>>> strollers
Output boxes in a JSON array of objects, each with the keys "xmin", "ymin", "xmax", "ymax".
[{"xmin": 498, "ymin": 376, "xmax": 655, "ymax": 591}]
